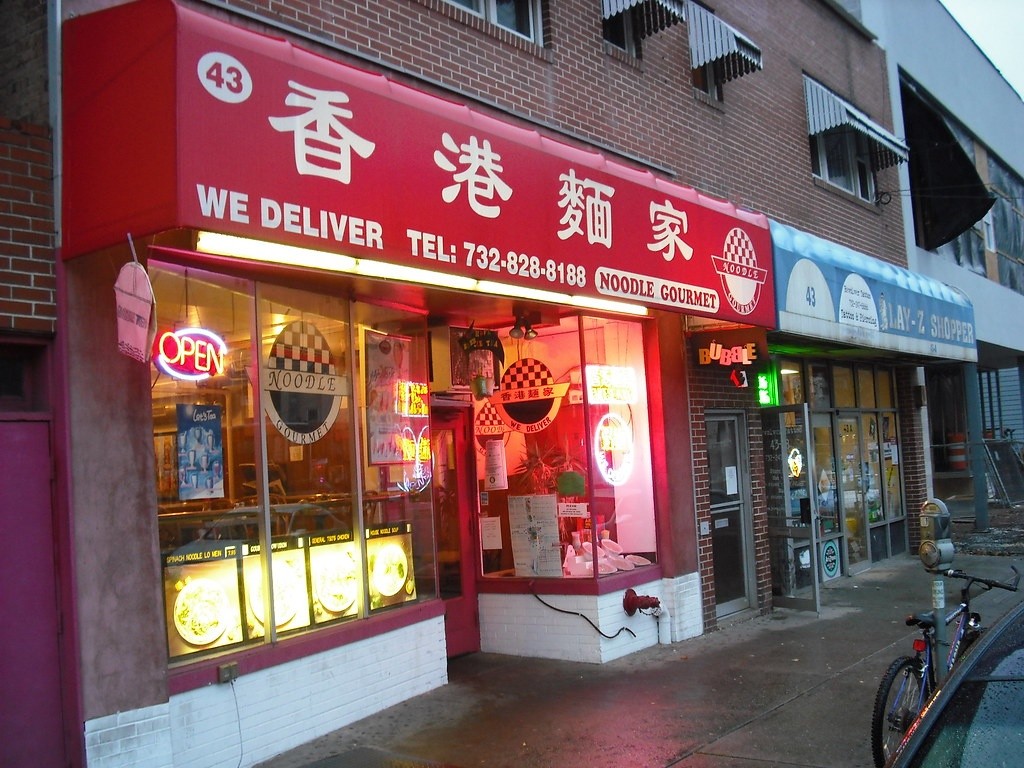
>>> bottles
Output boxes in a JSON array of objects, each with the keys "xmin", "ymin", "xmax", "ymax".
[{"xmin": 573, "ymin": 534, "xmax": 581, "ymax": 556}]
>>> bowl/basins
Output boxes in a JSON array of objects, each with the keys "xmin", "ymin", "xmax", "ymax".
[
  {"xmin": 583, "ymin": 542, "xmax": 605, "ymax": 561},
  {"xmin": 602, "ymin": 539, "xmax": 623, "ymax": 556}
]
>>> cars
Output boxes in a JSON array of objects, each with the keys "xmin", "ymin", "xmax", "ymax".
[
  {"xmin": 167, "ymin": 502, "xmax": 350, "ymax": 578},
  {"xmin": 885, "ymin": 599, "xmax": 1024, "ymax": 768}
]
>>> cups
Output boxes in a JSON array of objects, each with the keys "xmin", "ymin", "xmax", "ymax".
[
  {"xmin": 601, "ymin": 531, "xmax": 609, "ymax": 539},
  {"xmin": 191, "ymin": 475, "xmax": 198, "ymax": 488},
  {"xmin": 207, "ymin": 479, "xmax": 214, "ymax": 494}
]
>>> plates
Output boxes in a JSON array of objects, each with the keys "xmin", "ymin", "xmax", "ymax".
[
  {"xmin": 608, "ymin": 557, "xmax": 634, "ymax": 570},
  {"xmin": 590, "ymin": 563, "xmax": 617, "ymax": 574},
  {"xmin": 625, "ymin": 555, "xmax": 651, "ymax": 565}
]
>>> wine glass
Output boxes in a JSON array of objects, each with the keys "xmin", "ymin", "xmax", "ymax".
[
  {"xmin": 178, "ymin": 434, "xmax": 187, "ymax": 453},
  {"xmin": 207, "ymin": 436, "xmax": 213, "ymax": 452},
  {"xmin": 187, "ymin": 450, "xmax": 196, "ymax": 469},
  {"xmin": 179, "ymin": 469, "xmax": 187, "ymax": 487},
  {"xmin": 212, "ymin": 464, "xmax": 219, "ymax": 480},
  {"xmin": 201, "ymin": 456, "xmax": 208, "ymax": 472},
  {"xmin": 194, "ymin": 429, "xmax": 202, "ymax": 445}
]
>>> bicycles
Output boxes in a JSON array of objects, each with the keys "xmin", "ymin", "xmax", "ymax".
[{"xmin": 870, "ymin": 564, "xmax": 1020, "ymax": 768}]
